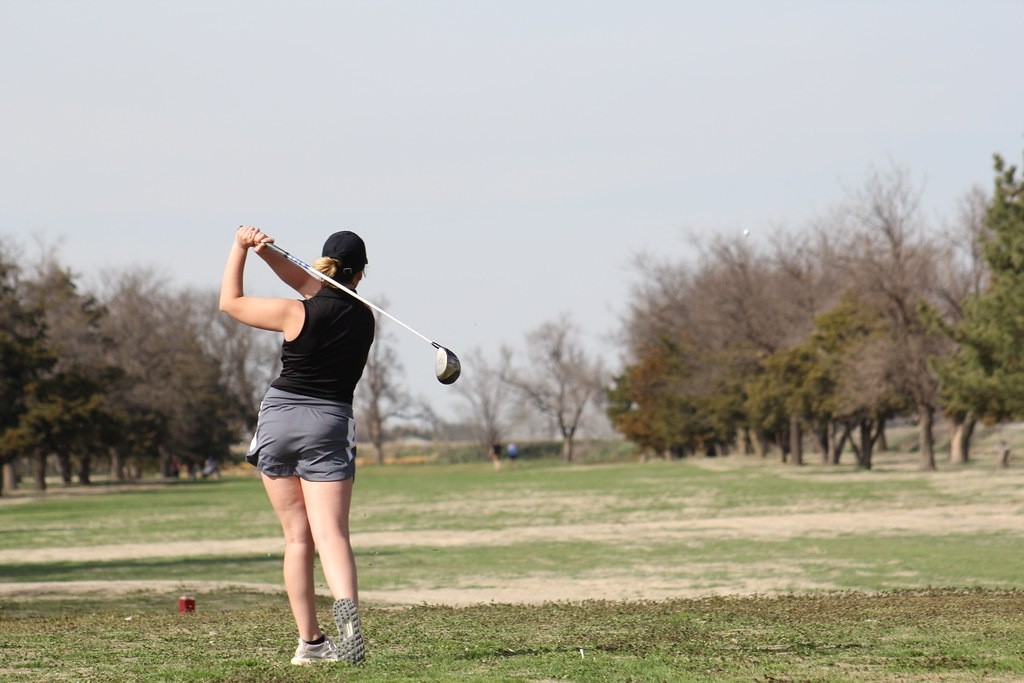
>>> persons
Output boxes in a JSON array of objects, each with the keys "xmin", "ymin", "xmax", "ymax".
[
  {"xmin": 219, "ymin": 224, "xmax": 377, "ymax": 670},
  {"xmin": 489, "ymin": 438, "xmax": 503, "ymax": 472},
  {"xmin": 507, "ymin": 441, "xmax": 518, "ymax": 470}
]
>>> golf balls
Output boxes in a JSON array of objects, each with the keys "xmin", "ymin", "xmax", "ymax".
[{"xmin": 631, "ymin": 402, "xmax": 639, "ymax": 410}]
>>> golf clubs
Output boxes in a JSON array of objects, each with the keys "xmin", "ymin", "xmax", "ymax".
[{"xmin": 237, "ymin": 224, "xmax": 461, "ymax": 385}]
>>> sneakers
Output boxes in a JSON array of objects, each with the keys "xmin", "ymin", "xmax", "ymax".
[
  {"xmin": 289, "ymin": 638, "xmax": 339, "ymax": 665},
  {"xmin": 332, "ymin": 598, "xmax": 367, "ymax": 666}
]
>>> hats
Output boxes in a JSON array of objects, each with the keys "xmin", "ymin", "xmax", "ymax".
[{"xmin": 322, "ymin": 231, "xmax": 368, "ymax": 275}]
